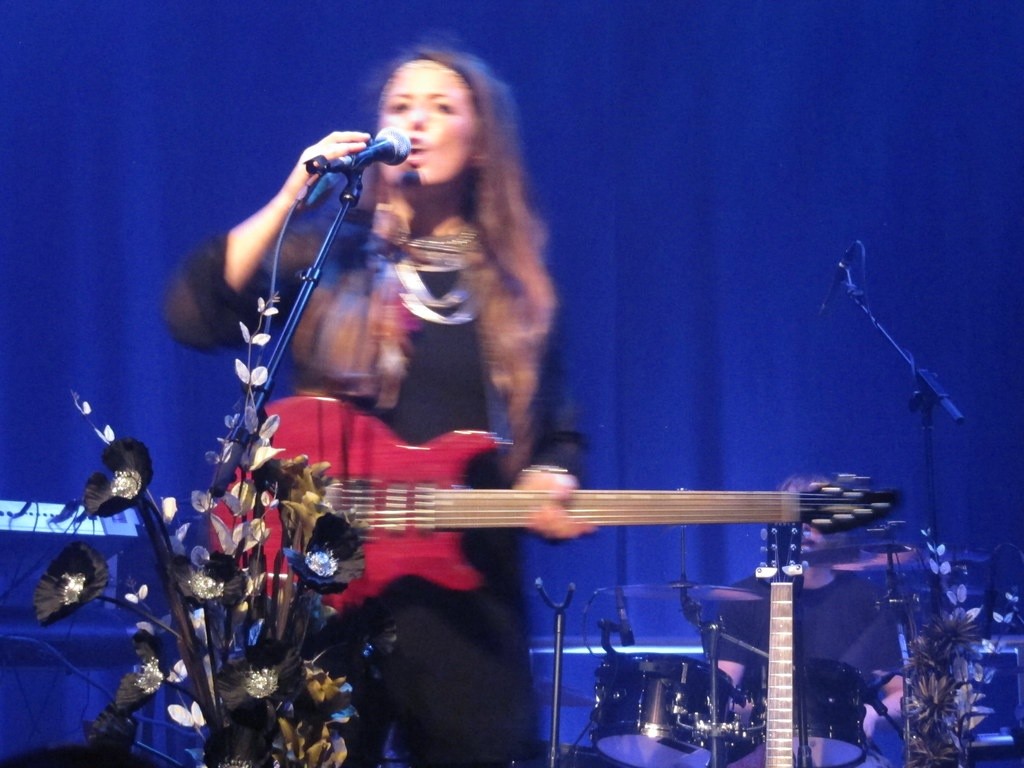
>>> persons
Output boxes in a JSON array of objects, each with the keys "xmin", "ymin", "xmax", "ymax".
[
  {"xmin": 169, "ymin": 49, "xmax": 584, "ymax": 767},
  {"xmin": 708, "ymin": 474, "xmax": 923, "ymax": 768}
]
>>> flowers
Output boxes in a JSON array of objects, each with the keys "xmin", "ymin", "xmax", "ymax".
[
  {"xmin": 894, "ymin": 527, "xmax": 1024, "ymax": 768},
  {"xmin": 31, "ymin": 285, "xmax": 365, "ymax": 768}
]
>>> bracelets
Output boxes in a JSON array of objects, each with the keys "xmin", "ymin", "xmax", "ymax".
[{"xmin": 524, "ymin": 465, "xmax": 570, "ymax": 473}]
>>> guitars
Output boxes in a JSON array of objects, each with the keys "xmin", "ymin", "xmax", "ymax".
[{"xmin": 209, "ymin": 393, "xmax": 901, "ymax": 636}]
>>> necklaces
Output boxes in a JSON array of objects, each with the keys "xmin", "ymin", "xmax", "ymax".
[{"xmin": 397, "ymin": 217, "xmax": 480, "ymax": 324}]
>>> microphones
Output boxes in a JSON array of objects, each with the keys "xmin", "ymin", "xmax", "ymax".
[
  {"xmin": 817, "ymin": 240, "xmax": 856, "ymax": 320},
  {"xmin": 613, "ymin": 586, "xmax": 634, "ymax": 646},
  {"xmin": 327, "ymin": 126, "xmax": 411, "ymax": 171}
]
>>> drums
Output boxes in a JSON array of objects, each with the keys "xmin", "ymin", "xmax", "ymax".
[
  {"xmin": 741, "ymin": 660, "xmax": 860, "ymax": 767},
  {"xmin": 590, "ymin": 652, "xmax": 733, "ymax": 768}
]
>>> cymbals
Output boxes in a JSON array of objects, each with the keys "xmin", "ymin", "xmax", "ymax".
[{"xmin": 593, "ymin": 584, "xmax": 760, "ymax": 602}]
